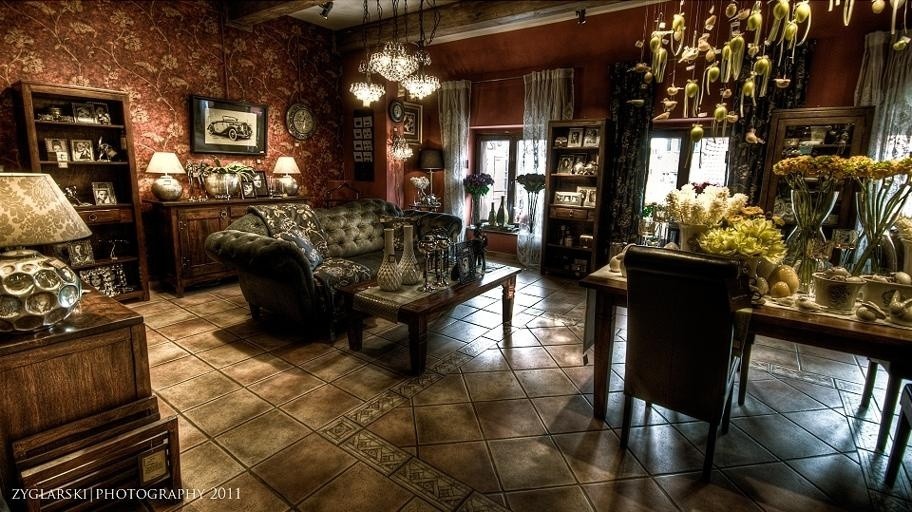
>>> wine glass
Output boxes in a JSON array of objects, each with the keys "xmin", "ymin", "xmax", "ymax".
[{"xmin": 637, "ymin": 218, "xmax": 654, "ymax": 246}]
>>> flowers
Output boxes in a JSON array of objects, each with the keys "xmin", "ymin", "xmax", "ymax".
[
  {"xmin": 409, "ymin": 176, "xmax": 430, "ymax": 195},
  {"xmin": 462, "ymin": 172, "xmax": 495, "ymax": 220},
  {"xmin": 662, "ymin": 154, "xmax": 912, "ymax": 283},
  {"xmin": 517, "ymin": 173, "xmax": 545, "ymax": 231}
]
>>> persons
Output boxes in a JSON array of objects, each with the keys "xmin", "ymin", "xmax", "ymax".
[
  {"xmin": 573, "ymin": 156, "xmax": 581, "ymax": 167},
  {"xmin": 72, "ymin": 243, "xmax": 90, "ymax": 265},
  {"xmin": 89, "ymin": 274, "xmax": 105, "ymax": 291},
  {"xmin": 94, "ymin": 104, "xmax": 106, "ymax": 126},
  {"xmin": 571, "ymin": 131, "xmax": 580, "ymax": 146},
  {"xmin": 55, "ymin": 243, "xmax": 72, "ymax": 265},
  {"xmin": 243, "ymin": 182, "xmax": 256, "ymax": 197},
  {"xmin": 75, "ymin": 106, "xmax": 91, "ymax": 124},
  {"xmin": 560, "ymin": 158, "xmax": 570, "ymax": 174},
  {"xmin": 52, "ymin": 140, "xmax": 65, "ymax": 161},
  {"xmin": 253, "ymin": 174, "xmax": 267, "ymax": 195},
  {"xmin": 97, "ymin": 190, "xmax": 111, "ymax": 204},
  {"xmin": 75, "ymin": 142, "xmax": 89, "ymax": 159}
]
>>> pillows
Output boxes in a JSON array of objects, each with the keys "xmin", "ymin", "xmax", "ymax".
[
  {"xmin": 273, "ymin": 230, "xmax": 323, "ymax": 271},
  {"xmin": 379, "ymin": 214, "xmax": 423, "ymax": 250}
]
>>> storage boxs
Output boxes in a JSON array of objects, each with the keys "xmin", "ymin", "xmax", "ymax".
[{"xmin": 578, "ymin": 232, "xmax": 594, "ymax": 250}]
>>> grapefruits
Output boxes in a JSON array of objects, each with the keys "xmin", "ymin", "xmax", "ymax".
[{"xmin": 771, "ymin": 264, "xmax": 800, "ymax": 292}]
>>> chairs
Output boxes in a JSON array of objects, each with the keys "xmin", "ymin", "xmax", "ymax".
[{"xmin": 621, "ymin": 246, "xmax": 754, "ymax": 477}]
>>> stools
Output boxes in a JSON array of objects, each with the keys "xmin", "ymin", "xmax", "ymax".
[
  {"xmin": 734, "ymin": 332, "xmax": 757, "ymax": 408},
  {"xmin": 882, "ymin": 382, "xmax": 911, "ymax": 489},
  {"xmin": 857, "ymin": 353, "xmax": 912, "ymax": 451}
]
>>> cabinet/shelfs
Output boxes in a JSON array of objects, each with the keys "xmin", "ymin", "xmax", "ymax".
[
  {"xmin": 10, "ymin": 79, "xmax": 151, "ymax": 305},
  {"xmin": 758, "ymin": 104, "xmax": 877, "ymax": 261},
  {"xmin": 0, "ymin": 280, "xmax": 187, "ymax": 512},
  {"xmin": 539, "ymin": 118, "xmax": 614, "ymax": 276}
]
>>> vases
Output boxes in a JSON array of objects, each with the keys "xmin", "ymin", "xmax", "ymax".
[
  {"xmin": 471, "ymin": 193, "xmax": 481, "ymax": 225},
  {"xmin": 525, "ymin": 193, "xmax": 537, "ymax": 232},
  {"xmin": 376, "ymin": 226, "xmax": 404, "ymax": 290},
  {"xmin": 398, "ymin": 223, "xmax": 421, "ymax": 284},
  {"xmin": 497, "ymin": 195, "xmax": 509, "ymax": 226},
  {"xmin": 488, "ymin": 202, "xmax": 497, "ymax": 227}
]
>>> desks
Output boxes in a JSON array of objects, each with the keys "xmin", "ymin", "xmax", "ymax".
[
  {"xmin": 466, "ymin": 223, "xmax": 542, "ymax": 257},
  {"xmin": 335, "ymin": 256, "xmax": 521, "ymax": 373},
  {"xmin": 153, "ymin": 198, "xmax": 312, "ymax": 297},
  {"xmin": 408, "ymin": 203, "xmax": 442, "ymax": 213},
  {"xmin": 580, "ymin": 256, "xmax": 912, "ymax": 489}
]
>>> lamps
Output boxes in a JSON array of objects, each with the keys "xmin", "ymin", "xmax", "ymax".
[
  {"xmin": 349, "ymin": 0, "xmax": 443, "ymax": 109},
  {"xmin": 271, "ymin": 156, "xmax": 303, "ymax": 195},
  {"xmin": 1, "ymin": 168, "xmax": 95, "ymax": 331},
  {"xmin": 317, "ymin": 2, "xmax": 336, "ymax": 20},
  {"xmin": 388, "ymin": 126, "xmax": 414, "ymax": 162},
  {"xmin": 575, "ymin": 8, "xmax": 588, "ymax": 26},
  {"xmin": 419, "ymin": 149, "xmax": 444, "ymax": 196},
  {"xmin": 146, "ymin": 151, "xmax": 187, "ymax": 201}
]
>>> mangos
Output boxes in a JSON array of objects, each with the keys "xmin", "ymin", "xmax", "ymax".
[
  {"xmin": 890, "ymin": 0, "xmax": 906, "ymax": 8},
  {"xmin": 872, "ymin": 0, "xmax": 886, "ymax": 14},
  {"xmin": 636, "ymin": 2, "xmax": 809, "ymax": 143}
]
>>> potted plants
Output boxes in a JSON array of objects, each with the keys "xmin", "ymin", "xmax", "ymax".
[{"xmin": 186, "ymin": 154, "xmax": 257, "ymax": 198}]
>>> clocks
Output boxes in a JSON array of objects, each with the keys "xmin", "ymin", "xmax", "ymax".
[
  {"xmin": 285, "ymin": 103, "xmax": 319, "ymax": 140},
  {"xmin": 389, "ymin": 100, "xmax": 407, "ymax": 125}
]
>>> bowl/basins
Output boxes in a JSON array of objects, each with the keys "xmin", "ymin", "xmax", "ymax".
[{"xmin": 810, "ymin": 272, "xmax": 911, "ymax": 315}]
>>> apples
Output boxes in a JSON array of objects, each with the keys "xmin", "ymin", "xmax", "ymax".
[{"xmin": 610, "ymin": 256, "xmax": 621, "ymax": 272}]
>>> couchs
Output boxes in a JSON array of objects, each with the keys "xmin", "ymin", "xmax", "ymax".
[{"xmin": 203, "ymin": 199, "xmax": 463, "ymax": 336}]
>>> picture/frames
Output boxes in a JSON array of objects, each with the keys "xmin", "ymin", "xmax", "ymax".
[
  {"xmin": 105, "ymin": 262, "xmax": 129, "ymax": 294},
  {"xmin": 452, "ymin": 241, "xmax": 477, "ymax": 285},
  {"xmin": 187, "ymin": 93, "xmax": 269, "ymax": 157},
  {"xmin": 239, "ymin": 168, "xmax": 270, "ymax": 198},
  {"xmin": 64, "ymin": 241, "xmax": 97, "ymax": 268},
  {"xmin": 76, "ymin": 266, "xmax": 116, "ymax": 301},
  {"xmin": 36, "ymin": 98, "xmax": 112, "ymax": 166},
  {"xmin": 89, "ymin": 178, "xmax": 120, "ymax": 207},
  {"xmin": 553, "ymin": 127, "xmax": 600, "ymax": 208},
  {"xmin": 403, "ymin": 102, "xmax": 424, "ymax": 146},
  {"xmin": 239, "ymin": 181, "xmax": 257, "ymax": 199}
]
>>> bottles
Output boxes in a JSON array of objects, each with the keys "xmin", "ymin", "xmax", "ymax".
[
  {"xmin": 488, "ymin": 196, "xmax": 509, "ymax": 227},
  {"xmin": 376, "ymin": 224, "xmax": 421, "ymax": 292},
  {"xmin": 424, "ymin": 235, "xmax": 451, "ymax": 289}
]
